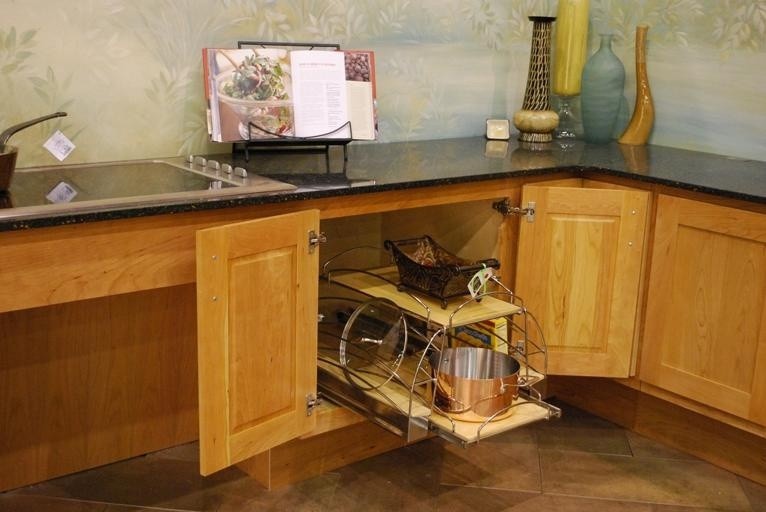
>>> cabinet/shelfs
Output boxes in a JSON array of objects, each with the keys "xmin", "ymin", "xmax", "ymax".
[
  {"xmin": 195, "ymin": 138, "xmax": 653, "ymax": 477},
  {"xmin": 556, "ymin": 140, "xmax": 766, "ymax": 485}
]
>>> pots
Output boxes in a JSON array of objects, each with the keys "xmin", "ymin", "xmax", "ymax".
[{"xmin": 428, "ymin": 346, "xmax": 522, "ymax": 425}]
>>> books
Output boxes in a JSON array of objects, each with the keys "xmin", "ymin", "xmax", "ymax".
[{"xmin": 202, "ymin": 47, "xmax": 379, "ymax": 143}]
